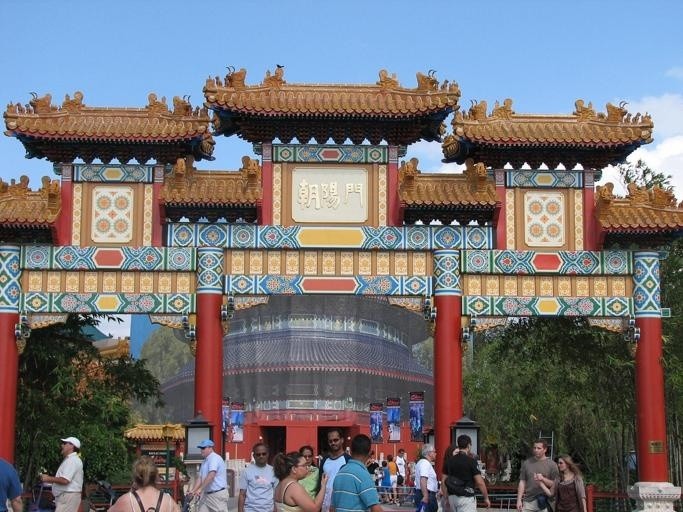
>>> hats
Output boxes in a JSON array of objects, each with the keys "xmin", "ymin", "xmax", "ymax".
[
  {"xmin": 60, "ymin": 437, "xmax": 80, "ymax": 449},
  {"xmin": 196, "ymin": 440, "xmax": 214, "ymax": 448}
]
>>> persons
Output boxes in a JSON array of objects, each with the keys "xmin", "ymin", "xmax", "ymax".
[
  {"xmin": 330, "ymin": 434, "xmax": 383, "ymax": 511},
  {"xmin": 414, "ymin": 443, "xmax": 438, "ymax": 511},
  {"xmin": 107, "ymin": 455, "xmax": 181, "ymax": 511},
  {"xmin": 1, "ymin": 457, "xmax": 23, "ymax": 511},
  {"xmin": 534, "ymin": 454, "xmax": 588, "ymax": 512},
  {"xmin": 441, "ymin": 435, "xmax": 489, "ymax": 511},
  {"xmin": 190, "ymin": 438, "xmax": 229, "ymax": 511},
  {"xmin": 343, "ymin": 446, "xmax": 352, "ymax": 457},
  {"xmin": 273, "ymin": 451, "xmax": 328, "ymax": 511},
  {"xmin": 237, "ymin": 442, "xmax": 279, "ymax": 511},
  {"xmin": 517, "ymin": 441, "xmax": 560, "ymax": 512},
  {"xmin": 314, "ymin": 428, "xmax": 351, "ymax": 511},
  {"xmin": 365, "ymin": 448, "xmax": 415, "ymax": 506},
  {"xmin": 40, "ymin": 436, "xmax": 83, "ymax": 511},
  {"xmin": 298, "ymin": 445, "xmax": 319, "ymax": 500}
]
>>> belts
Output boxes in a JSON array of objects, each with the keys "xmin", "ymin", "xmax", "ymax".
[{"xmin": 207, "ymin": 488, "xmax": 224, "ymax": 494}]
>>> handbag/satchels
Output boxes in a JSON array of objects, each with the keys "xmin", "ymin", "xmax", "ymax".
[
  {"xmin": 444, "ymin": 476, "xmax": 464, "ymax": 495},
  {"xmin": 396, "ymin": 476, "xmax": 402, "ymax": 484}
]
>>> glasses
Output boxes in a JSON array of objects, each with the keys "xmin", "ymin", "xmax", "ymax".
[
  {"xmin": 303, "ymin": 455, "xmax": 312, "ymax": 458},
  {"xmin": 327, "ymin": 439, "xmax": 338, "ymax": 443},
  {"xmin": 255, "ymin": 453, "xmax": 266, "ymax": 457}
]
